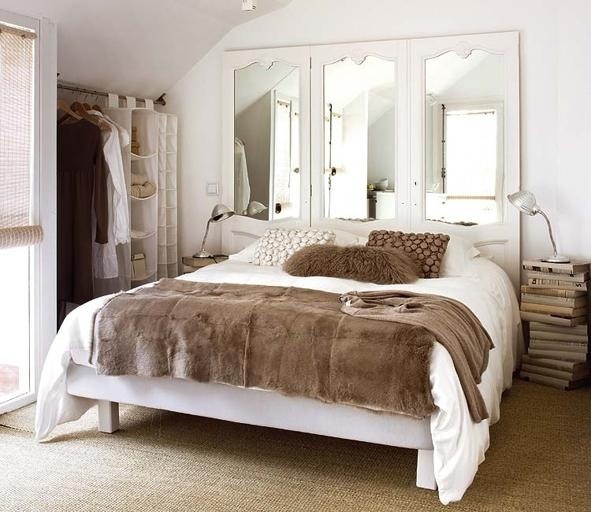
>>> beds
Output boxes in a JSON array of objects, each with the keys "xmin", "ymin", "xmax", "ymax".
[{"xmin": 33, "ymin": 256, "xmax": 525, "ymax": 505}]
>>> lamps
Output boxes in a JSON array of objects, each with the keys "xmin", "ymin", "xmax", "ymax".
[
  {"xmin": 508, "ymin": 190, "xmax": 572, "ymax": 262},
  {"xmin": 191, "ymin": 205, "xmax": 234, "ymax": 258}
]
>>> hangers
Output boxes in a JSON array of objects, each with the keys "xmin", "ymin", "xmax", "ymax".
[{"xmin": 59, "ymin": 80, "xmax": 123, "ymax": 132}]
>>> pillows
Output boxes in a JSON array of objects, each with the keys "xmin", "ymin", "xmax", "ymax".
[{"xmin": 227, "ymin": 227, "xmax": 480, "ymax": 284}]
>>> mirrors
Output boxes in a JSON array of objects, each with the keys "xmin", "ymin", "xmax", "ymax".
[
  {"xmin": 221, "ymin": 45, "xmax": 309, "ymax": 257},
  {"xmin": 409, "ymin": 30, "xmax": 521, "ymax": 296},
  {"xmin": 310, "ymin": 41, "xmax": 409, "ymax": 241}
]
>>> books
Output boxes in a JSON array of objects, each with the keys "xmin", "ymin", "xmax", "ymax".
[{"xmin": 518, "ymin": 257, "xmax": 591, "ymax": 390}]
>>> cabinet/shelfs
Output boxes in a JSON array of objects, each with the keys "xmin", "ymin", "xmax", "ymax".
[{"xmin": 93, "ymin": 108, "xmax": 177, "ymax": 298}]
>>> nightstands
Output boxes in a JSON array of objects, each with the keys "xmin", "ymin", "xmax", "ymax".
[{"xmin": 182, "ymin": 253, "xmax": 225, "ymax": 270}]
